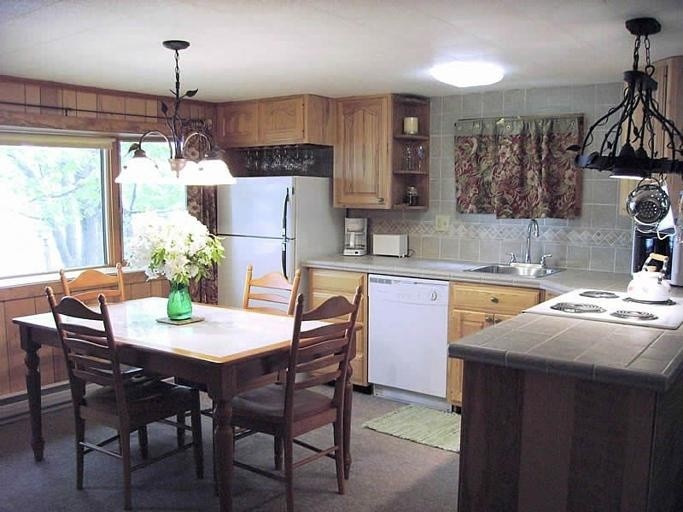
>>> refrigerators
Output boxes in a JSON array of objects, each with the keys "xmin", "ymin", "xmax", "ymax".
[{"xmin": 214, "ymin": 174, "xmax": 347, "ymax": 314}]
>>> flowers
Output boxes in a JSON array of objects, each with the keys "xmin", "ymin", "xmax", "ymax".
[{"xmin": 125, "ymin": 213, "xmax": 226, "ymax": 302}]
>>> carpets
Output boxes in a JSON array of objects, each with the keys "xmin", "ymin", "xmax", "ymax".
[{"xmin": 362, "ymin": 402, "xmax": 460, "ymax": 456}]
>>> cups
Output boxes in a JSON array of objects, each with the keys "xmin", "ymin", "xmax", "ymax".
[{"xmin": 403, "ymin": 116, "xmax": 418, "ymax": 134}]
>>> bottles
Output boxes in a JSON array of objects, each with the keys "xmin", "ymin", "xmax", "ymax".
[
  {"xmin": 402, "ymin": 142, "xmax": 426, "ymax": 171},
  {"xmin": 404, "ymin": 185, "xmax": 417, "ymax": 205}
]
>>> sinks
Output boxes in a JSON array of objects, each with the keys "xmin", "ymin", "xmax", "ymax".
[{"xmin": 461, "ymin": 262, "xmax": 569, "ymax": 281}]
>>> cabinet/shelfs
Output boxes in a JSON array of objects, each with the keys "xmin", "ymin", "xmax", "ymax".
[
  {"xmin": 332, "ymin": 95, "xmax": 429, "ymax": 209},
  {"xmin": 216, "ymin": 95, "xmax": 332, "ymax": 148},
  {"xmin": 305, "ymin": 265, "xmax": 364, "ymax": 400},
  {"xmin": 614, "ymin": 55, "xmax": 681, "ymax": 218},
  {"xmin": 445, "ymin": 275, "xmax": 542, "ymax": 435}
]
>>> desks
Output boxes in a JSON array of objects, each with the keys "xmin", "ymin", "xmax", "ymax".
[{"xmin": 12, "ymin": 293, "xmax": 364, "ymax": 496}]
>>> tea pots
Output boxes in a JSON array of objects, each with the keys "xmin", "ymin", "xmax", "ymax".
[{"xmin": 625, "ymin": 251, "xmax": 669, "ymax": 301}]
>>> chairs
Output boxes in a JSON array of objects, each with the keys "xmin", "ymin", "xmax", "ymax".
[
  {"xmin": 57, "ymin": 266, "xmax": 143, "ymax": 396},
  {"xmin": 176, "ymin": 264, "xmax": 302, "ymax": 454},
  {"xmin": 212, "ymin": 285, "xmax": 366, "ymax": 512},
  {"xmin": 46, "ymin": 285, "xmax": 205, "ymax": 511}
]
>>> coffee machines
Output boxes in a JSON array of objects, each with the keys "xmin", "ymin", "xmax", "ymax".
[{"xmin": 343, "ymin": 217, "xmax": 367, "ymax": 256}]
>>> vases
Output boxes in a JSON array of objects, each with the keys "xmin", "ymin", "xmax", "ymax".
[{"xmin": 166, "ymin": 275, "xmax": 193, "ymax": 320}]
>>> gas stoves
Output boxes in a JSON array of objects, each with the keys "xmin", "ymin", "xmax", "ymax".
[{"xmin": 520, "ymin": 287, "xmax": 682, "ymax": 330}]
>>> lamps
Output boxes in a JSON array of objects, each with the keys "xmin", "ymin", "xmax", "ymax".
[
  {"xmin": 565, "ymin": 15, "xmax": 682, "ymax": 185},
  {"xmin": 114, "ymin": 37, "xmax": 240, "ymax": 186},
  {"xmin": 430, "ymin": 59, "xmax": 503, "ymax": 89}
]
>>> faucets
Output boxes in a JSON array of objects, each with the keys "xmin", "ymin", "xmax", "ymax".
[{"xmin": 524, "ymin": 219, "xmax": 539, "ymax": 265}]
